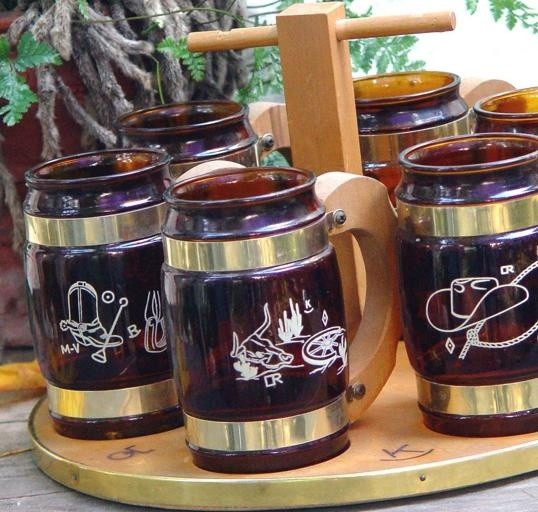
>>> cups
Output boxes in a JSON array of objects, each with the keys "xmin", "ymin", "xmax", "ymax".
[
  {"xmin": 25, "ymin": 146, "xmax": 246, "ymax": 445},
  {"xmin": 349, "ymin": 69, "xmax": 527, "ymax": 340},
  {"xmin": 476, "ymin": 82, "xmax": 538, "ymax": 136},
  {"xmin": 160, "ymin": 167, "xmax": 402, "ymax": 478},
  {"xmin": 110, "ymin": 98, "xmax": 290, "ymax": 190},
  {"xmin": 391, "ymin": 130, "xmax": 537, "ymax": 437}
]
